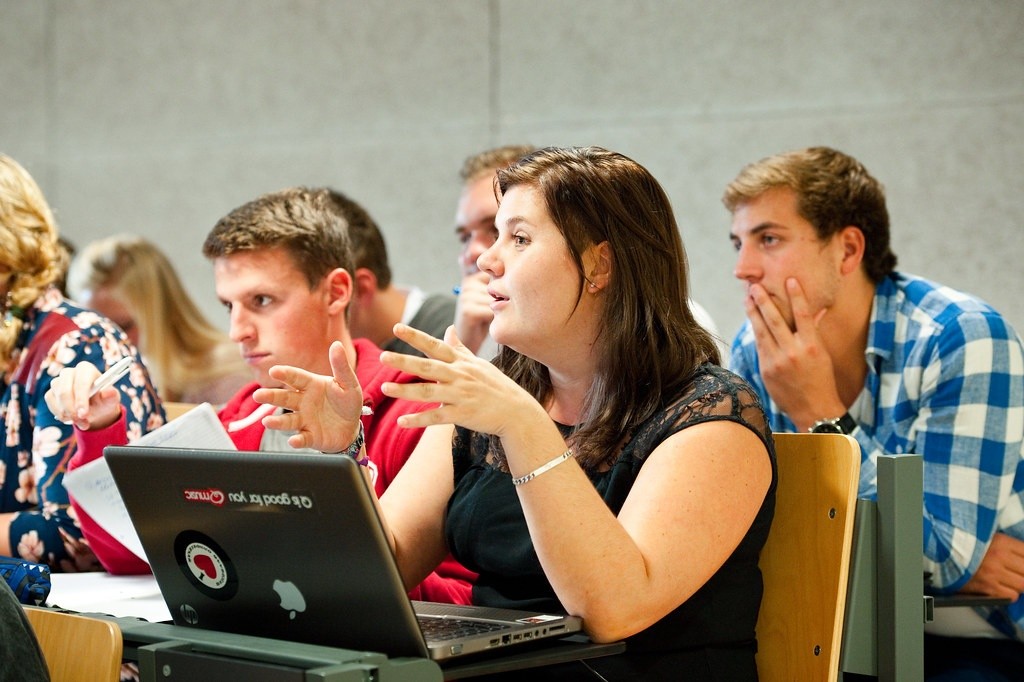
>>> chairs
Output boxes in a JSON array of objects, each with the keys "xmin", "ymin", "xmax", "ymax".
[{"xmin": 10, "ymin": 402, "xmax": 934, "ymax": 682}]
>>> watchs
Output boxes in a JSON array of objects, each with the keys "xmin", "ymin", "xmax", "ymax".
[{"xmin": 807, "ymin": 411, "xmax": 857, "ymax": 435}]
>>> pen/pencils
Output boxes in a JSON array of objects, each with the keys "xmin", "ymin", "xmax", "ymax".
[
  {"xmin": 453, "ymin": 288, "xmax": 460, "ymax": 294},
  {"xmin": 54, "ymin": 356, "xmax": 133, "ymax": 420}
]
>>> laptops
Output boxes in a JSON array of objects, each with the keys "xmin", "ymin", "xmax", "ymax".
[{"xmin": 103, "ymin": 446, "xmax": 582, "ymax": 662}]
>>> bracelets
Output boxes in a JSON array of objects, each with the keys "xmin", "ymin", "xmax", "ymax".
[
  {"xmin": 319, "ymin": 418, "xmax": 365, "ymax": 457},
  {"xmin": 512, "ymin": 449, "xmax": 573, "ymax": 485}
]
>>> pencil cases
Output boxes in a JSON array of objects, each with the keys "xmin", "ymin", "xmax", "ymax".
[{"xmin": 0, "ymin": 554, "xmax": 52, "ymax": 607}]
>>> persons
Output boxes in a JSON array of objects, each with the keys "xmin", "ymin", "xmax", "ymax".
[
  {"xmin": 723, "ymin": 146, "xmax": 1024, "ymax": 682},
  {"xmin": 66, "ymin": 232, "xmax": 253, "ymax": 405},
  {"xmin": 328, "ymin": 188, "xmax": 457, "ymax": 359},
  {"xmin": 455, "ymin": 140, "xmax": 720, "ymax": 364},
  {"xmin": 252, "ymin": 146, "xmax": 777, "ymax": 682},
  {"xmin": 0, "ymin": 152, "xmax": 167, "ymax": 572},
  {"xmin": 45, "ymin": 189, "xmax": 477, "ymax": 607}
]
